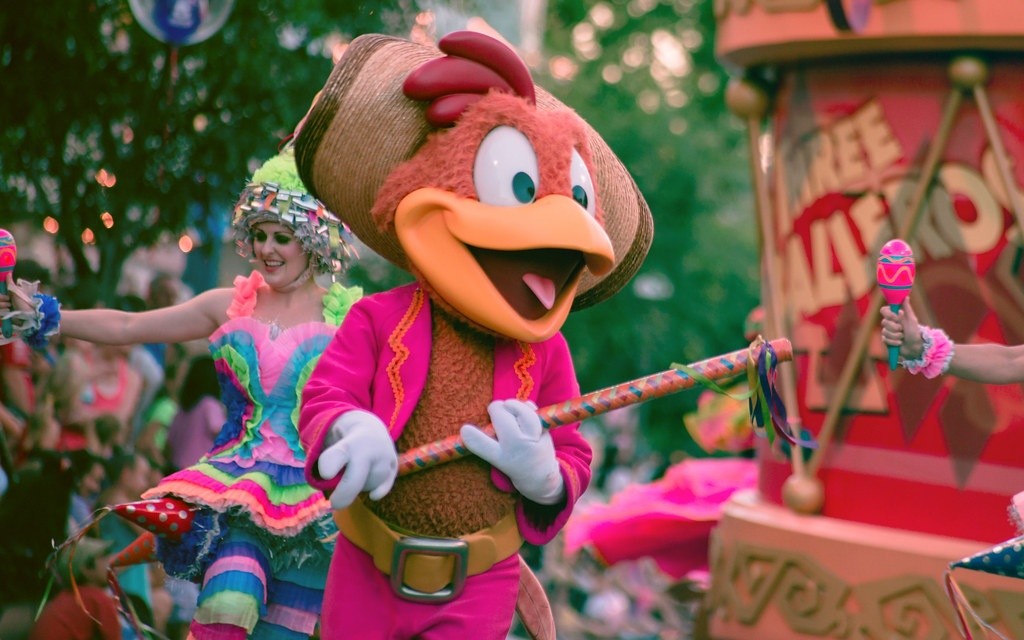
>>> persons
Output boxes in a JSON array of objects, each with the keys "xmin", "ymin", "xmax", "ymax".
[
  {"xmin": 879, "ymin": 231, "xmax": 1024, "ymax": 537},
  {"xmin": 0, "ymin": 219, "xmax": 681, "ymax": 640},
  {"xmin": 1, "ymin": 130, "xmax": 365, "ymax": 640}
]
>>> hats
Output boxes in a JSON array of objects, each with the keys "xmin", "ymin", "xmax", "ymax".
[
  {"xmin": 231, "ymin": 144, "xmax": 355, "ymax": 278},
  {"xmin": 59, "ymin": 534, "xmax": 111, "ymax": 579}
]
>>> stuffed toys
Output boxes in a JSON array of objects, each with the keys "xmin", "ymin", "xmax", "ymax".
[{"xmin": 292, "ymin": 17, "xmax": 655, "ymax": 640}]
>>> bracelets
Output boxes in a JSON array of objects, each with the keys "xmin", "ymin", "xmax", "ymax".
[
  {"xmin": 21, "ymin": 293, "xmax": 61, "ymax": 351},
  {"xmin": 902, "ymin": 324, "xmax": 954, "ymax": 379}
]
